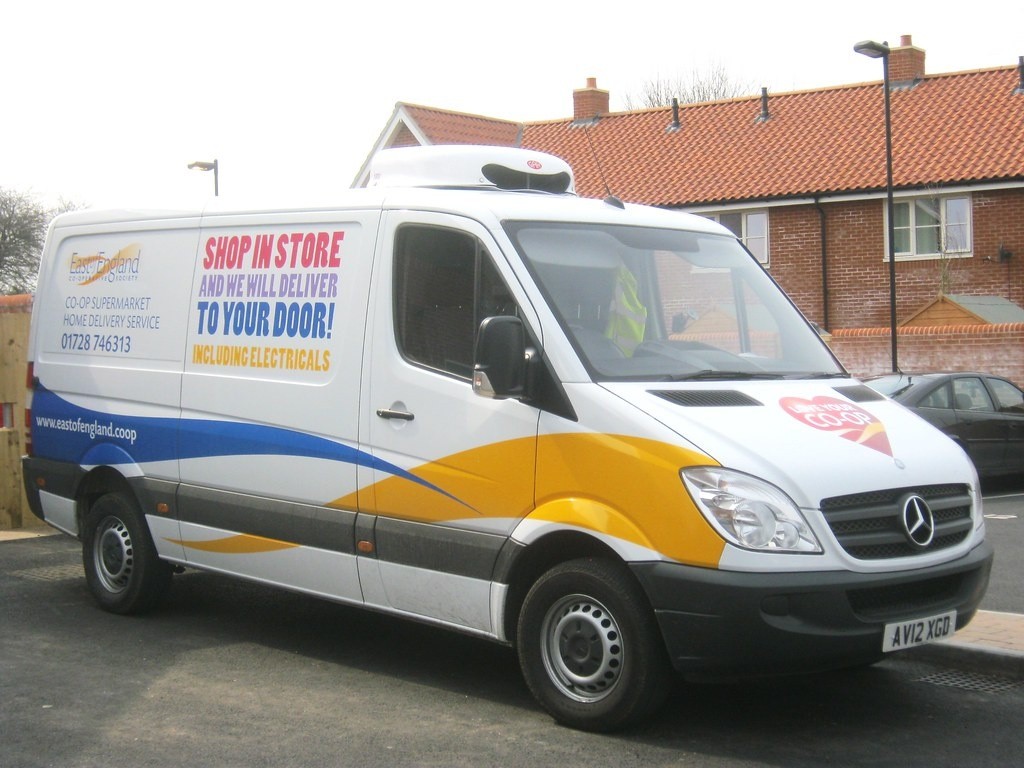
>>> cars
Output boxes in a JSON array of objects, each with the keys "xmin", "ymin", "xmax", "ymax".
[{"xmin": 861, "ymin": 372, "xmax": 1023, "ymax": 478}]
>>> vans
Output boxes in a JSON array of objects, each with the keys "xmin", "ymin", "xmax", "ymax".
[{"xmin": 23, "ymin": 145, "xmax": 996, "ymax": 731}]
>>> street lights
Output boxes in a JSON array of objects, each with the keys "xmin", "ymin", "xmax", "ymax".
[
  {"xmin": 187, "ymin": 160, "xmax": 218, "ymax": 197},
  {"xmin": 853, "ymin": 40, "xmax": 898, "ymax": 372}
]
